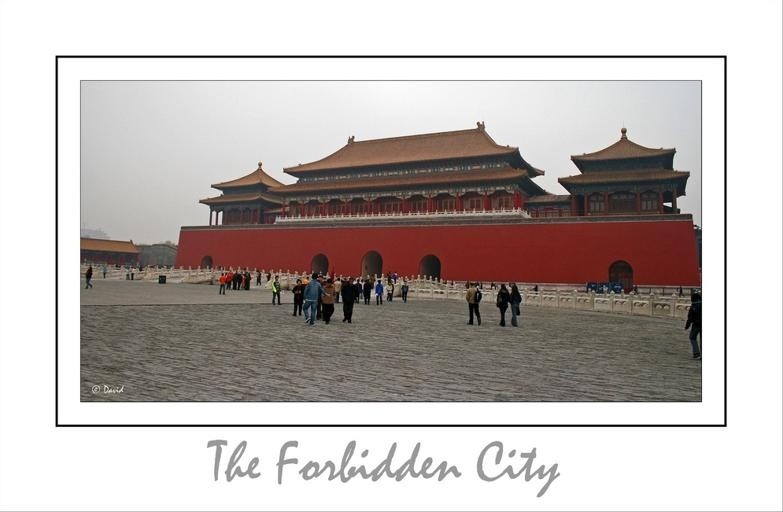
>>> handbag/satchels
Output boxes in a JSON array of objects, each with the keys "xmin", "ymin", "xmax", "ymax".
[{"xmin": 515, "ymin": 305, "xmax": 521, "ymax": 317}]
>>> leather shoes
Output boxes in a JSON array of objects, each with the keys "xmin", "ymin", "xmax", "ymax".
[
  {"xmin": 348, "ymin": 320, "xmax": 351, "ymax": 323},
  {"xmin": 342, "ymin": 317, "xmax": 347, "ymax": 322}
]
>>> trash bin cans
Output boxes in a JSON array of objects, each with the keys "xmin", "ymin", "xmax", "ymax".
[
  {"xmin": 126, "ymin": 274, "xmax": 129, "ymax": 280},
  {"xmin": 158, "ymin": 276, "xmax": 166, "ymax": 284},
  {"xmin": 131, "ymin": 273, "xmax": 134, "ymax": 280},
  {"xmin": 588, "ymin": 281, "xmax": 622, "ymax": 293}
]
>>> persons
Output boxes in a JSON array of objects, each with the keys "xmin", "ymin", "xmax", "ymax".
[
  {"xmin": 115, "ymin": 263, "xmax": 147, "ymax": 280},
  {"xmin": 634, "ymin": 283, "xmax": 638, "ymax": 294},
  {"xmin": 219, "ymin": 267, "xmax": 409, "ymax": 328},
  {"xmin": 685, "ymin": 293, "xmax": 701, "ymax": 361},
  {"xmin": 85, "ymin": 266, "xmax": 92, "ymax": 289},
  {"xmin": 101, "ymin": 265, "xmax": 107, "ymax": 279},
  {"xmin": 679, "ymin": 285, "xmax": 685, "ymax": 297},
  {"xmin": 465, "ymin": 279, "xmax": 522, "ymax": 327}
]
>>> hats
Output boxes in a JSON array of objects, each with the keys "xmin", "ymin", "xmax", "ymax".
[{"xmin": 312, "ymin": 272, "xmax": 319, "ymax": 279}]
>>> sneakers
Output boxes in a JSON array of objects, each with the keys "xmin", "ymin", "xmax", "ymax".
[
  {"xmin": 305, "ymin": 316, "xmax": 311, "ymax": 324},
  {"xmin": 690, "ymin": 352, "xmax": 701, "ymax": 360}
]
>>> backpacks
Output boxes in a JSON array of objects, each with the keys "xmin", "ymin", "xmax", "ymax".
[{"xmin": 475, "ymin": 291, "xmax": 482, "ymax": 303}]
